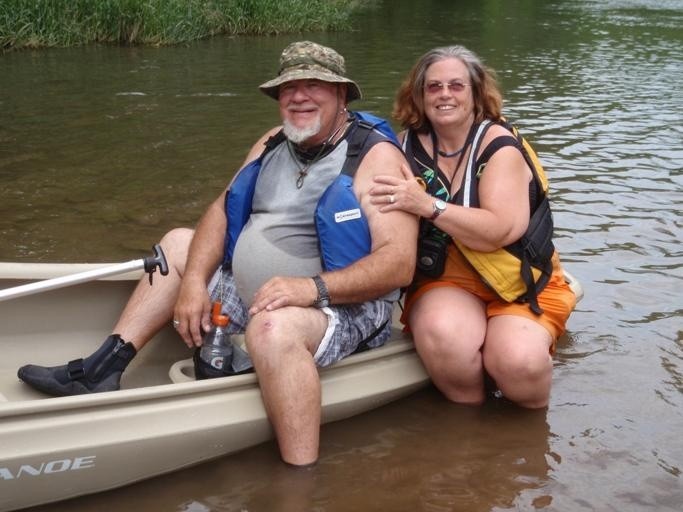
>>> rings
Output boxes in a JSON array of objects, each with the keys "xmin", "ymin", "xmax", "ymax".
[
  {"xmin": 173, "ymin": 320, "xmax": 180, "ymax": 325},
  {"xmin": 390, "ymin": 196, "xmax": 393, "ymax": 202}
]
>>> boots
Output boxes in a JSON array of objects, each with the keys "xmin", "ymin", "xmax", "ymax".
[{"xmin": 18, "ymin": 334, "xmax": 137, "ymax": 396}]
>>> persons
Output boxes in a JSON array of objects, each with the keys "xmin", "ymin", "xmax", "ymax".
[
  {"xmin": 367, "ymin": 44, "xmax": 585, "ymax": 414},
  {"xmin": 13, "ymin": 39, "xmax": 420, "ymax": 468}
]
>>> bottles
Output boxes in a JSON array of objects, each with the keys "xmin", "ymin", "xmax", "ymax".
[{"xmin": 198, "ymin": 314, "xmax": 232, "ymax": 371}]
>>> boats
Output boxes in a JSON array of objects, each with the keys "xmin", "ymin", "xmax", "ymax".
[{"xmin": 0, "ymin": 260, "xmax": 585, "ymax": 510}]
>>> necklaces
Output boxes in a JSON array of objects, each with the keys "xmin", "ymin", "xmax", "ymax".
[{"xmin": 438, "ymin": 146, "xmax": 464, "ymax": 158}]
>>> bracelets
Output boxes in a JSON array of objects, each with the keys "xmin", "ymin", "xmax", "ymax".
[
  {"xmin": 425, "ymin": 197, "xmax": 447, "ymax": 223},
  {"xmin": 311, "ymin": 274, "xmax": 330, "ymax": 309}
]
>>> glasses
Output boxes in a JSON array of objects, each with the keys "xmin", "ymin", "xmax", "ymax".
[{"xmin": 421, "ymin": 80, "xmax": 475, "ymax": 93}]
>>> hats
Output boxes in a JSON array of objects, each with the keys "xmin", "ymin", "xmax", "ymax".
[{"xmin": 258, "ymin": 40, "xmax": 363, "ymax": 104}]
